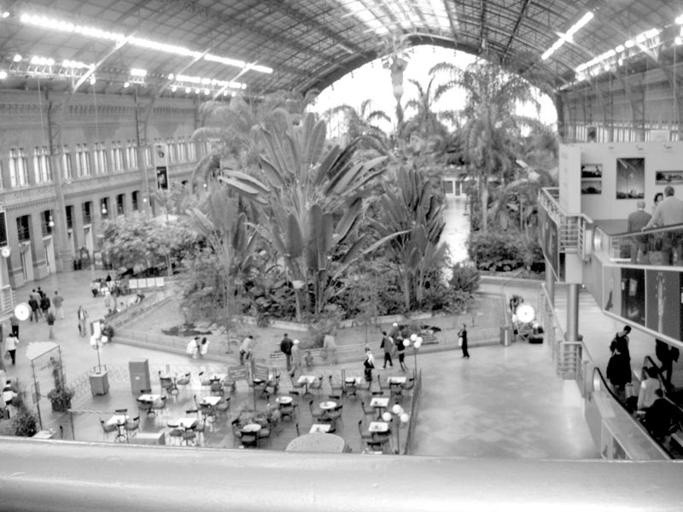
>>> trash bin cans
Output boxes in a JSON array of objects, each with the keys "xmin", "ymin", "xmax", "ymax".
[{"xmin": 500, "ymin": 326, "xmax": 512, "ymax": 347}]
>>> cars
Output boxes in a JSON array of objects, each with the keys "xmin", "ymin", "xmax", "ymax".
[{"xmin": 78, "ymin": 316, "xmax": 114, "ymax": 340}]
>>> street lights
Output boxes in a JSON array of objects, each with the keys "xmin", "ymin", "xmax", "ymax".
[
  {"xmin": 46, "ymin": 215, "xmax": 58, "ymax": 274},
  {"xmin": 142, "ymin": 194, "xmax": 148, "ymax": 215},
  {"xmin": 101, "ymin": 204, "xmax": 108, "ymax": 223},
  {"xmin": 403, "ymin": 333, "xmax": 423, "ymax": 378},
  {"xmin": 203, "ymin": 183, "xmax": 207, "ymax": 191},
  {"xmin": 89, "ymin": 331, "xmax": 108, "ymax": 377}
]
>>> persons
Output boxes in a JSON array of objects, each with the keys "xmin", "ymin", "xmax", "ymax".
[
  {"xmin": 232, "ymin": 418, "xmax": 243, "ymax": 438},
  {"xmin": 2, "ymin": 380, "xmax": 17, "ymax": 419},
  {"xmin": 458, "ymin": 324, "xmax": 470, "ymax": 358},
  {"xmin": 93, "ymin": 273, "xmax": 130, "ymax": 297},
  {"xmin": 187, "ymin": 337, "xmax": 209, "ymax": 359},
  {"xmin": 78, "ymin": 306, "xmax": 114, "ymax": 343},
  {"xmin": 362, "ymin": 322, "xmax": 408, "ymax": 381},
  {"xmin": 28, "ymin": 286, "xmax": 64, "ymax": 338},
  {"xmin": 239, "ymin": 335, "xmax": 253, "ymax": 364},
  {"xmin": 626, "ymin": 187, "xmax": 682, "ymax": 266},
  {"xmin": 280, "ymin": 333, "xmax": 300, "ymax": 372},
  {"xmin": 606, "ymin": 324, "xmax": 683, "ymax": 444},
  {"xmin": 6, "ymin": 333, "xmax": 19, "ymax": 363}
]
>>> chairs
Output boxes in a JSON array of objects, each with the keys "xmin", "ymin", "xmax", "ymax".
[
  {"xmin": 99, "ymin": 408, "xmax": 141, "ymax": 443},
  {"xmin": 193, "ymin": 394, "xmax": 230, "ymax": 424},
  {"xmin": 158, "ymin": 370, "xmax": 191, "ymax": 396},
  {"xmin": 135, "ymin": 389, "xmax": 167, "ymax": 415},
  {"xmin": 167, "ymin": 409, "xmax": 205, "ymax": 446},
  {"xmin": 231, "ymin": 364, "xmax": 413, "ymax": 455},
  {"xmin": 198, "ymin": 372, "xmax": 236, "ymax": 394}
]
